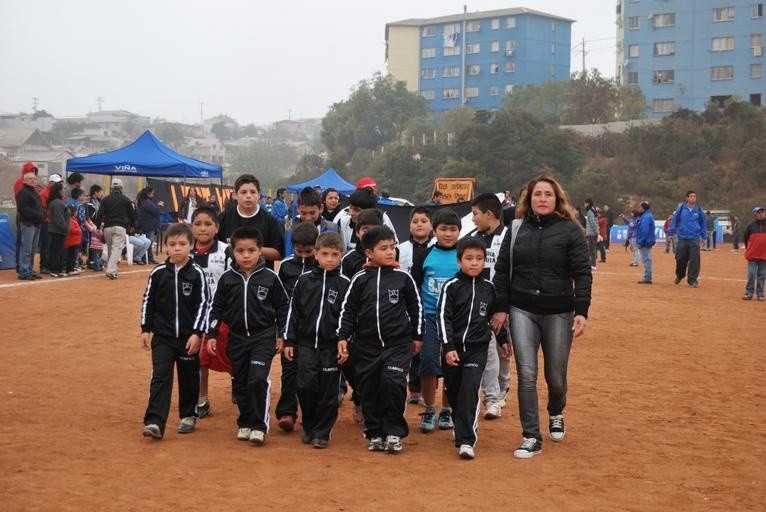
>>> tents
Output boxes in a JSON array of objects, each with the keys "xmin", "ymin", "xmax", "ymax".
[
  {"xmin": 287, "ymin": 167, "xmax": 357, "ymax": 196},
  {"xmin": 65, "ymin": 128, "xmax": 222, "ymax": 221}
]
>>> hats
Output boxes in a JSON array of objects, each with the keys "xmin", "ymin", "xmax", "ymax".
[
  {"xmin": 48, "ymin": 174, "xmax": 63, "ymax": 183},
  {"xmin": 110, "ymin": 179, "xmax": 122, "ymax": 188}
]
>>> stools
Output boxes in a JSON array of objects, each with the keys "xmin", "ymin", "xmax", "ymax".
[{"xmin": 120, "ymin": 233, "xmax": 149, "ymax": 268}]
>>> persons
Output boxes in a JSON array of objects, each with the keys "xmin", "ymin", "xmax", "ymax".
[
  {"xmin": 14, "ymin": 163, "xmax": 165, "ymax": 278},
  {"xmin": 620, "ymin": 201, "xmax": 656, "ymax": 283},
  {"xmin": 744, "ymin": 206, "xmax": 766, "ymax": 301},
  {"xmin": 584, "ymin": 198, "xmax": 613, "ymax": 271},
  {"xmin": 140, "ymin": 173, "xmax": 592, "ymax": 457},
  {"xmin": 731, "ymin": 216, "xmax": 741, "ymax": 250},
  {"xmin": 664, "ymin": 191, "xmax": 718, "ymax": 288}
]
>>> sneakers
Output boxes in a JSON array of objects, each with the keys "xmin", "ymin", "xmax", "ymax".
[
  {"xmin": 418, "ymin": 412, "xmax": 436, "ymax": 431},
  {"xmin": 513, "ymin": 439, "xmax": 542, "ymax": 459},
  {"xmin": 384, "ymin": 435, "xmax": 402, "ymax": 452},
  {"xmin": 459, "ymin": 445, "xmax": 474, "ymax": 459},
  {"xmin": 367, "ymin": 437, "xmax": 384, "ymax": 451},
  {"xmin": 548, "ymin": 415, "xmax": 565, "ymax": 441},
  {"xmin": 142, "ymin": 424, "xmax": 162, "ymax": 438},
  {"xmin": 438, "ymin": 410, "xmax": 453, "ymax": 430},
  {"xmin": 352, "ymin": 406, "xmax": 364, "ymax": 426},
  {"xmin": 177, "ymin": 400, "xmax": 209, "ymax": 433},
  {"xmin": 482, "ymin": 388, "xmax": 509, "ymax": 419},
  {"xmin": 236, "ymin": 415, "xmax": 327, "ymax": 449}
]
